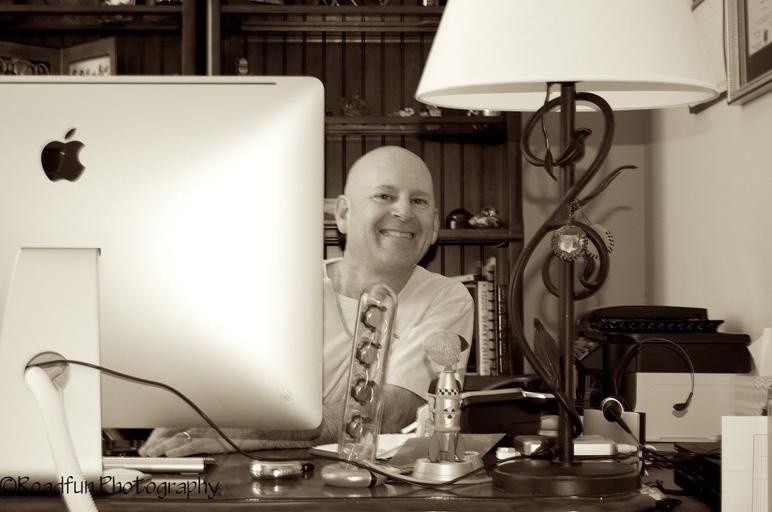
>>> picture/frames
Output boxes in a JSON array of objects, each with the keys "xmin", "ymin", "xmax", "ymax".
[
  {"xmin": 721, "ymin": 1, "xmax": 772, "ymax": 110},
  {"xmin": 687, "ymin": 0, "xmax": 731, "ymax": 115}
]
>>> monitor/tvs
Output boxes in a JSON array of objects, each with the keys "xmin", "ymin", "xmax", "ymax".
[{"xmin": 0, "ymin": 75, "xmax": 325, "ymax": 494}]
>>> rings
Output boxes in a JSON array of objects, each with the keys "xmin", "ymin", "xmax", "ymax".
[{"xmin": 182, "ymin": 431, "xmax": 192, "ymax": 440}]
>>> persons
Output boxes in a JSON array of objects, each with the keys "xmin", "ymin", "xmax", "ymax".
[{"xmin": 138, "ymin": 145, "xmax": 475, "ymax": 457}]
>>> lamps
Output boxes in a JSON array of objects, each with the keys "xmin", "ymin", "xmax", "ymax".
[{"xmin": 411, "ymin": 0, "xmax": 718, "ymax": 502}]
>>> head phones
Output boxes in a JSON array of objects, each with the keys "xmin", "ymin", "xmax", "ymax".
[{"xmin": 613, "ymin": 338, "xmax": 695, "ymax": 411}]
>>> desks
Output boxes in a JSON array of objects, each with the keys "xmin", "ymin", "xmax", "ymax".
[{"xmin": 0, "ymin": 438, "xmax": 771, "ymax": 511}]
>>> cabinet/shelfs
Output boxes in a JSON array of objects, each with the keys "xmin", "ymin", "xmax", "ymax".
[
  {"xmin": 208, "ymin": 1, "xmax": 523, "ymax": 386},
  {"xmin": 573, "ymin": 331, "xmax": 754, "ymax": 412},
  {"xmin": 1, "ymin": 1, "xmax": 200, "ymax": 79}
]
